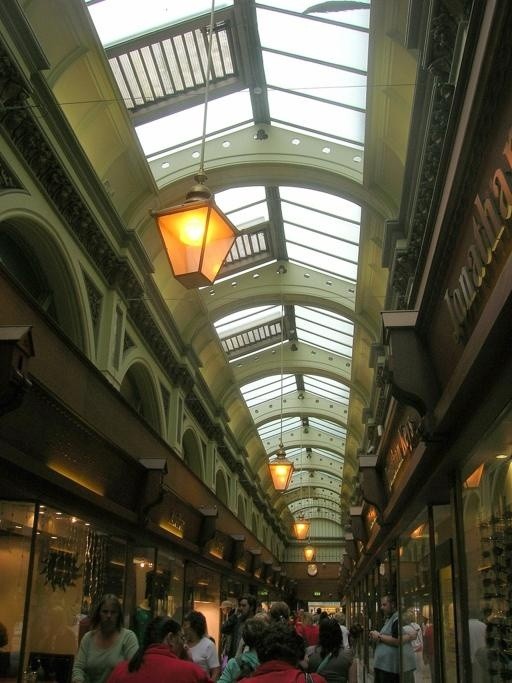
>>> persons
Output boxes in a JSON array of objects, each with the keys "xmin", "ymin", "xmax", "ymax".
[
  {"xmin": 71, "ymin": 593, "xmax": 400, "ymax": 682},
  {"xmin": 401, "ymin": 608, "xmax": 487, "ymax": 683}
]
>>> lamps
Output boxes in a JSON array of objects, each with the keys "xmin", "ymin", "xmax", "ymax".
[
  {"xmin": 302, "ymin": 469, "xmax": 315, "ymax": 561},
  {"xmin": 290, "ymin": 417, "xmax": 311, "ymax": 541},
  {"xmin": 266, "ymin": 269, "xmax": 294, "ymax": 493},
  {"xmin": 149, "ymin": 0, "xmax": 240, "ymax": 290}
]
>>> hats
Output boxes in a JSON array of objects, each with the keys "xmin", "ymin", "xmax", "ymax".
[{"xmin": 220, "ymin": 601, "xmax": 233, "ymax": 609}]
